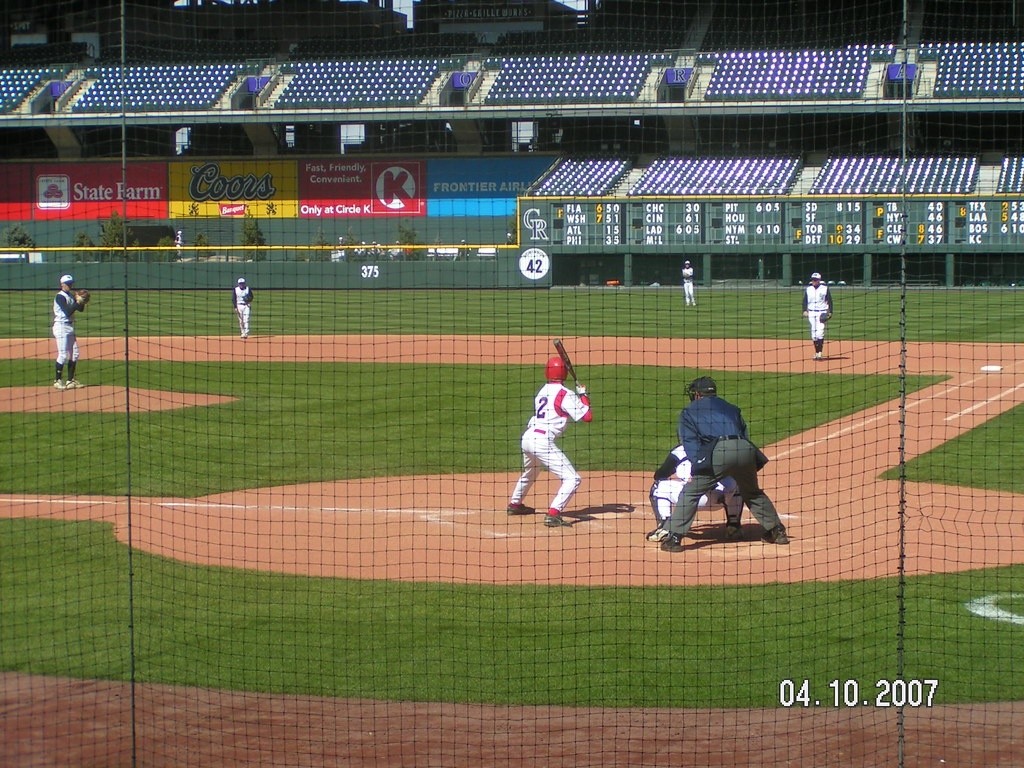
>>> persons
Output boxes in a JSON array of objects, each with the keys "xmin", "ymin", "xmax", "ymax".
[
  {"xmin": 458, "ymin": 239, "xmax": 471, "ymax": 261},
  {"xmin": 232, "ymin": 277, "xmax": 253, "ymax": 338},
  {"xmin": 681, "ymin": 260, "xmax": 696, "ymax": 306},
  {"xmin": 506, "ymin": 356, "xmax": 593, "ymax": 526},
  {"xmin": 52, "ymin": 275, "xmax": 87, "ymax": 390},
  {"xmin": 645, "ymin": 377, "xmax": 788, "ymax": 552},
  {"xmin": 338, "ymin": 236, "xmax": 419, "ymax": 261},
  {"xmin": 802, "ymin": 272, "xmax": 833, "ymax": 360},
  {"xmin": 175, "ymin": 231, "xmax": 183, "ymax": 261},
  {"xmin": 506, "ymin": 233, "xmax": 514, "ymax": 245}
]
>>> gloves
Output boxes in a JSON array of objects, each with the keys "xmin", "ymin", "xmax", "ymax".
[{"xmin": 576, "ymin": 384, "xmax": 587, "ymax": 394}]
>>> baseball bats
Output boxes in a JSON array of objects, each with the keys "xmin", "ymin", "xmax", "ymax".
[{"xmin": 553, "ymin": 338, "xmax": 581, "ymax": 387}]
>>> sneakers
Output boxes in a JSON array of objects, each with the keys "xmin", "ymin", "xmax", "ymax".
[
  {"xmin": 507, "ymin": 504, "xmax": 535, "ymax": 515},
  {"xmin": 544, "ymin": 516, "xmax": 570, "ymax": 526}
]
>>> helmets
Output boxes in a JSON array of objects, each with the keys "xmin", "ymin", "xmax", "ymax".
[{"xmin": 545, "ymin": 357, "xmax": 567, "ymax": 380}]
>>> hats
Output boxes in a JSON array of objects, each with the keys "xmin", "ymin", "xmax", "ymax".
[
  {"xmin": 692, "ymin": 378, "xmax": 716, "ymax": 392},
  {"xmin": 238, "ymin": 278, "xmax": 245, "ymax": 284},
  {"xmin": 685, "ymin": 261, "xmax": 690, "ymax": 264},
  {"xmin": 60, "ymin": 275, "xmax": 75, "ymax": 284},
  {"xmin": 810, "ymin": 273, "xmax": 821, "ymax": 279}
]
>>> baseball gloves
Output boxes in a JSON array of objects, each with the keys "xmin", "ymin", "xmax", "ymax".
[
  {"xmin": 78, "ymin": 289, "xmax": 90, "ymax": 304},
  {"xmin": 242, "ymin": 294, "xmax": 251, "ymax": 302},
  {"xmin": 820, "ymin": 313, "xmax": 828, "ymax": 323},
  {"xmin": 685, "ymin": 274, "xmax": 690, "ymax": 280}
]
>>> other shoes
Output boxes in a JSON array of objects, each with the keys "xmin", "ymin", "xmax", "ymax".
[
  {"xmin": 646, "ymin": 526, "xmax": 671, "ymax": 541},
  {"xmin": 686, "ymin": 303, "xmax": 689, "ymax": 306},
  {"xmin": 726, "ymin": 524, "xmax": 741, "ymax": 539},
  {"xmin": 661, "ymin": 535, "xmax": 682, "ymax": 551},
  {"xmin": 54, "ymin": 380, "xmax": 66, "ymax": 388},
  {"xmin": 761, "ymin": 530, "xmax": 788, "ymax": 544},
  {"xmin": 66, "ymin": 379, "xmax": 85, "ymax": 389},
  {"xmin": 692, "ymin": 302, "xmax": 696, "ymax": 306},
  {"xmin": 813, "ymin": 353, "xmax": 821, "ymax": 360}
]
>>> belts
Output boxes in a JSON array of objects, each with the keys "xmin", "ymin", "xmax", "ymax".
[
  {"xmin": 535, "ymin": 429, "xmax": 546, "ymax": 434},
  {"xmin": 809, "ymin": 310, "xmax": 825, "ymax": 311},
  {"xmin": 718, "ymin": 435, "xmax": 743, "ymax": 440}
]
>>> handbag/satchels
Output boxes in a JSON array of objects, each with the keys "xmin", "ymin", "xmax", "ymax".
[
  {"xmin": 749, "ymin": 442, "xmax": 769, "ymax": 470},
  {"xmin": 690, "ymin": 438, "xmax": 717, "ymax": 476}
]
